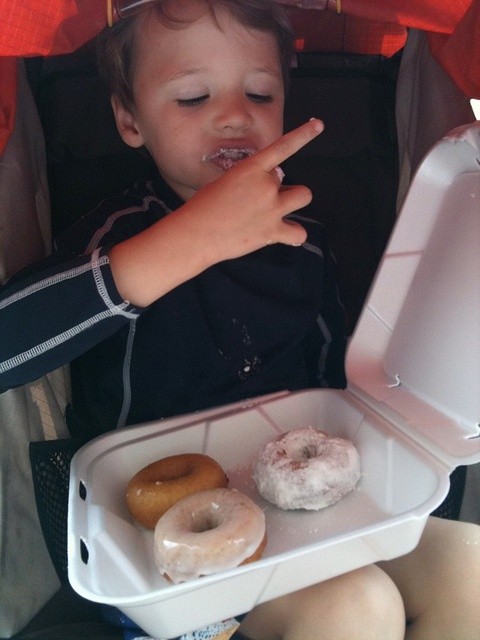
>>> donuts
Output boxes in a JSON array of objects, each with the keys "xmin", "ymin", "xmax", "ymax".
[
  {"xmin": 127, "ymin": 453, "xmax": 229, "ymax": 528},
  {"xmin": 253, "ymin": 425, "xmax": 362, "ymax": 511},
  {"xmin": 154, "ymin": 488, "xmax": 267, "ymax": 585}
]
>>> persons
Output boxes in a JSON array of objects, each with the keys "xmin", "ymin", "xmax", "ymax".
[{"xmin": 0, "ymin": 1, "xmax": 480, "ymax": 640}]
